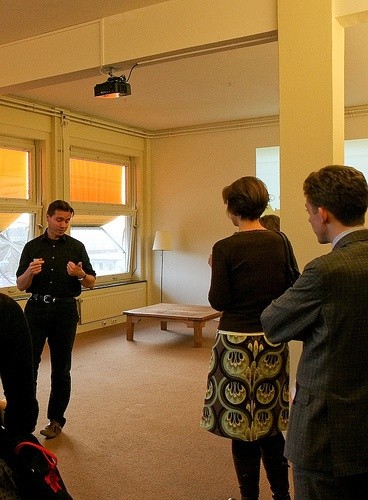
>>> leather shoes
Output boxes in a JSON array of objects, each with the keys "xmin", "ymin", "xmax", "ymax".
[{"xmin": 40, "ymin": 420, "xmax": 62, "ymax": 438}]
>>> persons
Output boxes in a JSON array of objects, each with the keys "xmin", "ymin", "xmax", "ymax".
[
  {"xmin": 16, "ymin": 200, "xmax": 96, "ymax": 438},
  {"xmin": 259, "ymin": 215, "xmax": 280, "ymax": 234},
  {"xmin": 261, "ymin": 165, "xmax": 367, "ymax": 500},
  {"xmin": 0, "ymin": 293, "xmax": 40, "ymax": 499},
  {"xmin": 201, "ymin": 177, "xmax": 300, "ymax": 500}
]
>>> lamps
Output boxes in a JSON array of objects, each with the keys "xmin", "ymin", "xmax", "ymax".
[{"xmin": 152, "ymin": 231, "xmax": 174, "ymax": 304}]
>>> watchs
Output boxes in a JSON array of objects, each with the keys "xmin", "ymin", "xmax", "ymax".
[{"xmin": 77, "ymin": 272, "xmax": 87, "ymax": 282}]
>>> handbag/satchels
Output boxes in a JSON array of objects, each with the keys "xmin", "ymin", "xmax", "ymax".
[
  {"xmin": 15, "ymin": 441, "xmax": 62, "ymax": 493},
  {"xmin": 277, "ymin": 231, "xmax": 301, "ymax": 286}
]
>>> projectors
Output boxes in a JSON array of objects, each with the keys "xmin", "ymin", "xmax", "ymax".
[{"xmin": 94, "ymin": 74, "xmax": 131, "ymax": 98}]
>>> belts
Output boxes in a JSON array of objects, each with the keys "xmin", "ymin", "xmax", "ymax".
[{"xmin": 33, "ymin": 294, "xmax": 73, "ymax": 303}]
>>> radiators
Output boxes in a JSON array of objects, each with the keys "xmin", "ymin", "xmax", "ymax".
[{"xmin": 80, "ymin": 288, "xmax": 147, "ymax": 325}]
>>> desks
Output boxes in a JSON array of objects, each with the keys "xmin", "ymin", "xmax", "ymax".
[{"xmin": 123, "ymin": 303, "xmax": 224, "ymax": 349}]
